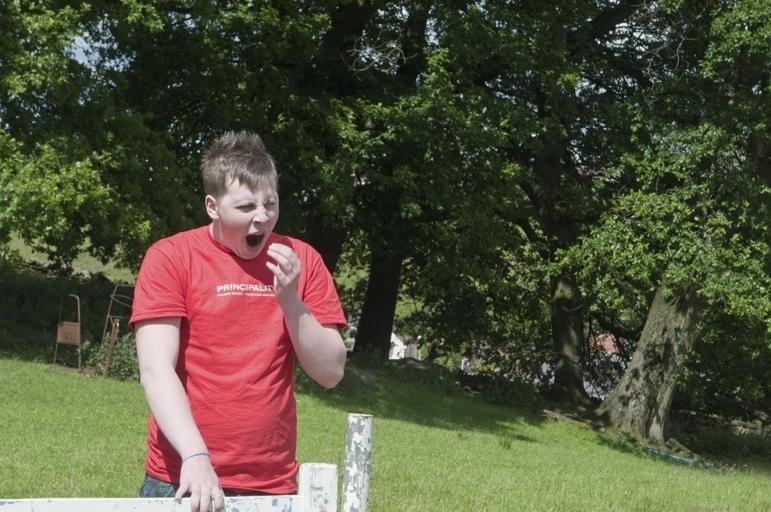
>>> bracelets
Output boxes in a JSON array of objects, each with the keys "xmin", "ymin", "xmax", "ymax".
[{"xmin": 179, "ymin": 452, "xmax": 212, "ymax": 465}]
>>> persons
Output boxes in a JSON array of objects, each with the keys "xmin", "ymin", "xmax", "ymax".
[{"xmin": 128, "ymin": 130, "xmax": 347, "ymax": 511}]
